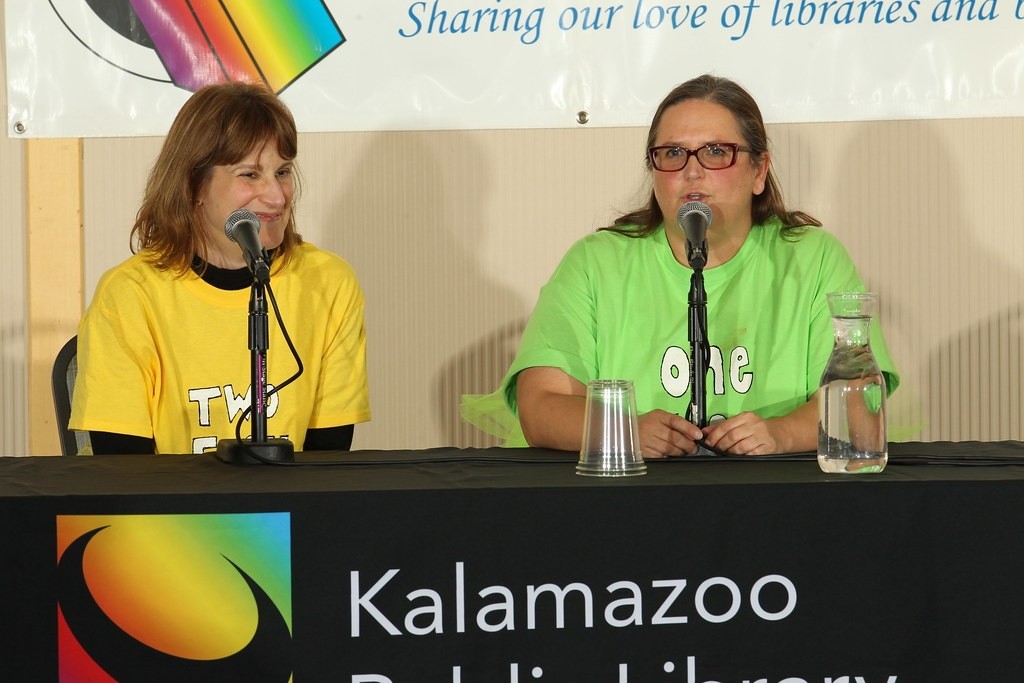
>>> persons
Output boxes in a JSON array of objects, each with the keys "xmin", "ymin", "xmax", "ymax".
[
  {"xmin": 460, "ymin": 75, "xmax": 928, "ymax": 458},
  {"xmin": 67, "ymin": 82, "xmax": 372, "ymax": 457}
]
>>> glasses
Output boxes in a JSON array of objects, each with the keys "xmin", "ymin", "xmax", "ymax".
[{"xmin": 648, "ymin": 143, "xmax": 760, "ymax": 172}]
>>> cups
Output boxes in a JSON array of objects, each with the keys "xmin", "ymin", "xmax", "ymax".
[{"xmin": 576, "ymin": 379, "xmax": 648, "ymax": 479}]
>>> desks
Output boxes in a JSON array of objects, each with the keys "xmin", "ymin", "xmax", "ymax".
[{"xmin": 1, "ymin": 448, "xmax": 1024, "ymax": 683}]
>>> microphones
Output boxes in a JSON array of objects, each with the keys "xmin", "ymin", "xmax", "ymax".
[
  {"xmin": 224, "ymin": 208, "xmax": 272, "ymax": 286},
  {"xmin": 676, "ymin": 201, "xmax": 713, "ymax": 266}
]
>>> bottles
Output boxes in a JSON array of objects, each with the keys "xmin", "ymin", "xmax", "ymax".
[{"xmin": 817, "ymin": 292, "xmax": 889, "ymax": 475}]
